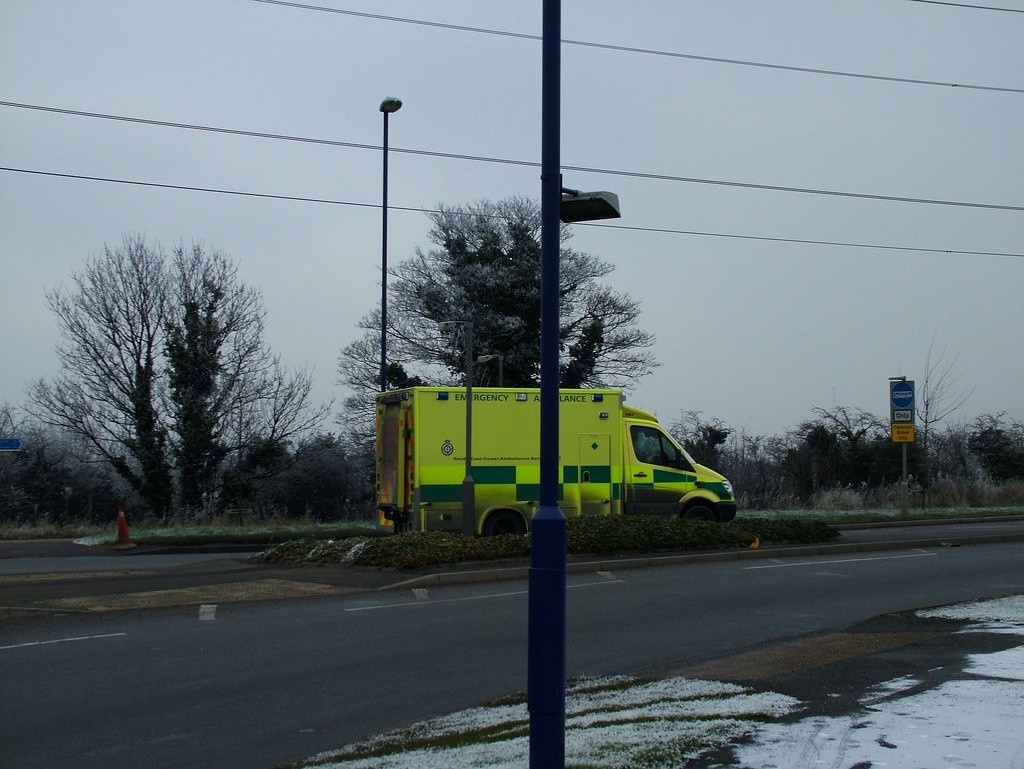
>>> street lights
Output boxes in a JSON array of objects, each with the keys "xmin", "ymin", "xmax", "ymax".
[
  {"xmin": 888, "ymin": 376, "xmax": 908, "ymax": 513},
  {"xmin": 528, "ymin": 185, "xmax": 620, "ymax": 769},
  {"xmin": 380, "ymin": 96, "xmax": 404, "ymax": 395},
  {"xmin": 437, "ymin": 320, "xmax": 476, "ymax": 539},
  {"xmin": 475, "ymin": 353, "xmax": 503, "ymax": 389}
]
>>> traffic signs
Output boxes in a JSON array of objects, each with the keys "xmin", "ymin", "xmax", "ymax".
[{"xmin": 0, "ymin": 438, "xmax": 23, "ymax": 451}]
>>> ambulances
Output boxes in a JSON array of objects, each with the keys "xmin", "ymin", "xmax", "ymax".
[{"xmin": 376, "ymin": 386, "xmax": 736, "ymax": 538}]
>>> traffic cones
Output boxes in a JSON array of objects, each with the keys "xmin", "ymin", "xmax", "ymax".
[{"xmin": 112, "ymin": 507, "xmax": 137, "ymax": 550}]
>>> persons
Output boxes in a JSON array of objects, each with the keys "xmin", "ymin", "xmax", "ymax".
[{"xmin": 632, "ymin": 431, "xmax": 661, "ymax": 464}]
